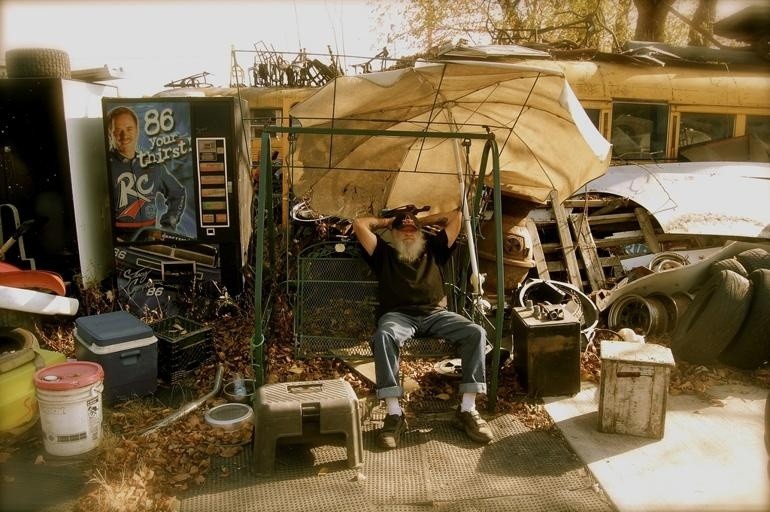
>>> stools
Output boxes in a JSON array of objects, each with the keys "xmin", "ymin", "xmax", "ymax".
[
  {"xmin": 249, "ymin": 379, "xmax": 364, "ymax": 476},
  {"xmin": 595, "ymin": 338, "xmax": 675, "ymax": 442}
]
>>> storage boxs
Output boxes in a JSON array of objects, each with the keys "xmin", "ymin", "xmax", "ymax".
[{"xmin": 72, "ymin": 310, "xmax": 155, "ymax": 408}]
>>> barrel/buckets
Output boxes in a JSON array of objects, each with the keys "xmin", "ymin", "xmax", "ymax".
[
  {"xmin": 33, "ymin": 361, "xmax": 105, "ymax": 458},
  {"xmin": 476, "ymin": 214, "xmax": 534, "ymax": 292}
]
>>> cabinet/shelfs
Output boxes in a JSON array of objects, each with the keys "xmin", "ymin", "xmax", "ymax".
[{"xmin": 510, "ymin": 302, "xmax": 582, "ymax": 402}]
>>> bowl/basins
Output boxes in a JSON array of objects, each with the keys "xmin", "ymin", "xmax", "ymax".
[{"xmin": 222, "ymin": 378, "xmax": 260, "ymax": 405}]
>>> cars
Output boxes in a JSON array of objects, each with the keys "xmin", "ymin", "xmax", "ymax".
[{"xmin": 112, "ymin": 227, "xmax": 218, "ymax": 295}]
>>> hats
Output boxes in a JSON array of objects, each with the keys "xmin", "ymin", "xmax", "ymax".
[{"xmin": 393, "ymin": 213, "xmax": 419, "ymax": 230}]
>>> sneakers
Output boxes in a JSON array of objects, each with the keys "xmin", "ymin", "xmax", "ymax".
[
  {"xmin": 379, "ymin": 411, "xmax": 408, "ymax": 448},
  {"xmin": 452, "ymin": 405, "xmax": 493, "ymax": 442}
]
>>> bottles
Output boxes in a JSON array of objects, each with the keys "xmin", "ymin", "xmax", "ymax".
[{"xmin": 230, "ymin": 371, "xmax": 246, "ymax": 396}]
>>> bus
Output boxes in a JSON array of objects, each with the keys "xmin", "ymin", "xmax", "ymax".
[
  {"xmin": 145, "ymin": 77, "xmax": 326, "ymax": 172},
  {"xmin": 434, "ymin": 42, "xmax": 770, "ymax": 309}
]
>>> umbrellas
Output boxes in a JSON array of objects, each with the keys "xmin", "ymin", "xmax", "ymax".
[{"xmin": 281, "ymin": 55, "xmax": 614, "ymax": 337}]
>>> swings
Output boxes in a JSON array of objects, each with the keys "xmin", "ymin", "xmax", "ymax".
[{"xmin": 286, "ymin": 130, "xmax": 482, "ymax": 359}]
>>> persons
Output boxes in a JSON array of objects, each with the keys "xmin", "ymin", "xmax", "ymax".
[
  {"xmin": 106, "ymin": 106, "xmax": 187, "ymax": 248},
  {"xmin": 351, "ymin": 205, "xmax": 494, "ymax": 450}
]
